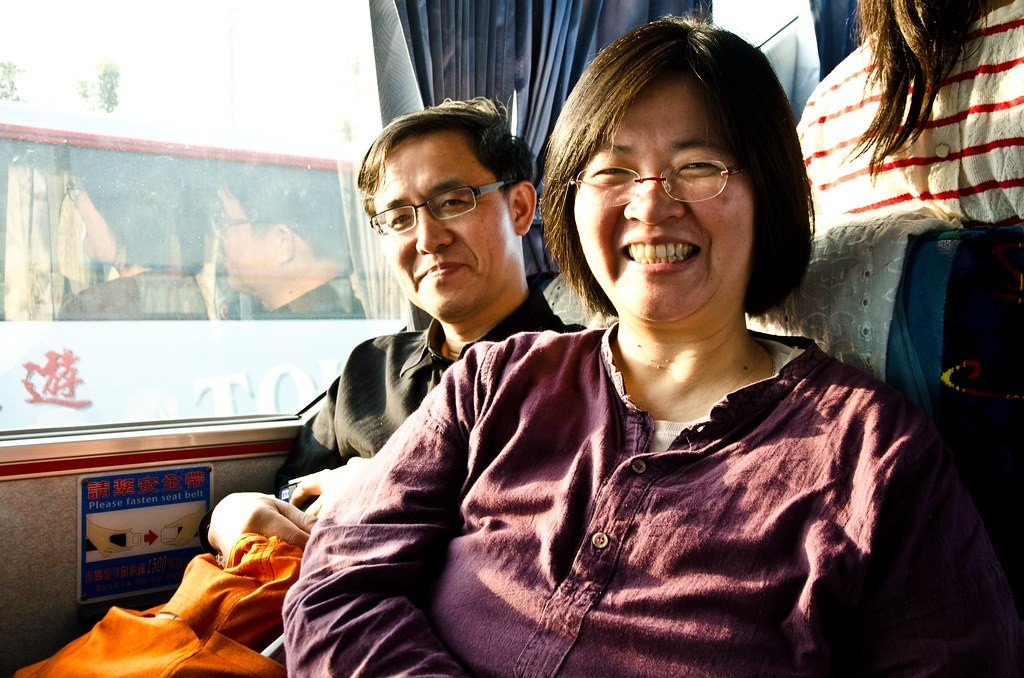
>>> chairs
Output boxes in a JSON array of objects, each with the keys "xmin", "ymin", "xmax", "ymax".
[{"xmin": 538, "ymin": 211, "xmax": 1024, "ymax": 603}]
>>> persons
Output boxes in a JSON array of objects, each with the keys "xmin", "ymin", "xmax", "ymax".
[
  {"xmin": 212, "ymin": 158, "xmax": 368, "ymax": 321},
  {"xmin": 281, "ymin": 14, "xmax": 1024, "ymax": 678},
  {"xmin": 55, "ymin": 145, "xmax": 211, "ymax": 321},
  {"xmin": 792, "ymin": 0, "xmax": 1024, "ymax": 240},
  {"xmin": 198, "ymin": 97, "xmax": 585, "ymax": 571}
]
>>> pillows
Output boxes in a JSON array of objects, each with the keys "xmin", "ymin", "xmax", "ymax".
[{"xmin": 795, "ymin": 0, "xmax": 1024, "ymax": 222}]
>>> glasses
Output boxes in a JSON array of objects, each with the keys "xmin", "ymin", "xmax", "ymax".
[
  {"xmin": 369, "ymin": 178, "xmax": 520, "ymax": 236},
  {"xmin": 570, "ymin": 159, "xmax": 747, "ymax": 207},
  {"xmin": 63, "ymin": 170, "xmax": 87, "ymax": 200},
  {"xmin": 210, "ymin": 209, "xmax": 283, "ymax": 238}
]
